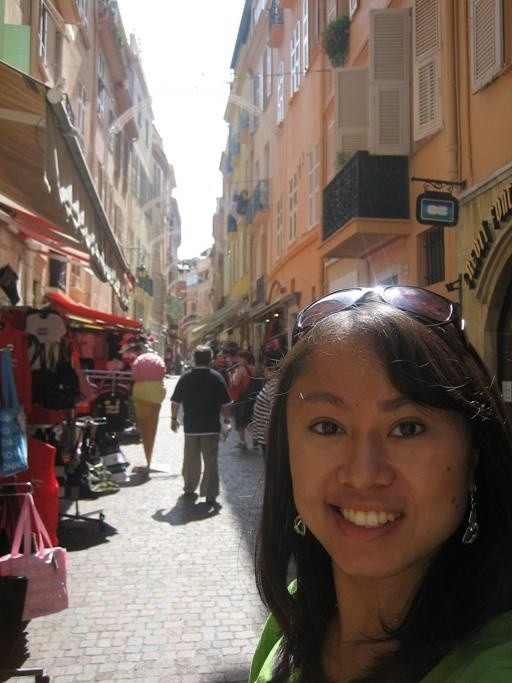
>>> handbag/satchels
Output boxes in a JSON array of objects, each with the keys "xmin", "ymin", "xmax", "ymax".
[
  {"xmin": 243, "ymin": 365, "xmax": 265, "ymax": 399},
  {"xmin": 96, "ymin": 399, "xmax": 129, "ymax": 431},
  {"xmin": 57, "ymin": 433, "xmax": 132, "ymax": 499},
  {"xmin": 1, "ymin": 530, "xmax": 68, "ymax": 644},
  {"xmin": 1, "ymin": 405, "xmax": 28, "ymax": 478},
  {"xmin": 31, "ymin": 365, "xmax": 97, "ymax": 409}
]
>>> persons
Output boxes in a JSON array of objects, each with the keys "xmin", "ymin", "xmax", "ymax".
[
  {"xmin": 248, "ymin": 285, "xmax": 512, "ymax": 683},
  {"xmin": 132, "ymin": 335, "xmax": 280, "ymax": 503}
]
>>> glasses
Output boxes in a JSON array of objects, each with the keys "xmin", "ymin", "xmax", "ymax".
[{"xmin": 292, "ymin": 286, "xmax": 469, "ymax": 352}]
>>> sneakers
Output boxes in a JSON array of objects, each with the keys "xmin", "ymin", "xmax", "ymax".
[
  {"xmin": 235, "ymin": 439, "xmax": 261, "ymax": 448},
  {"xmin": 186, "ymin": 489, "xmax": 215, "ymax": 504}
]
